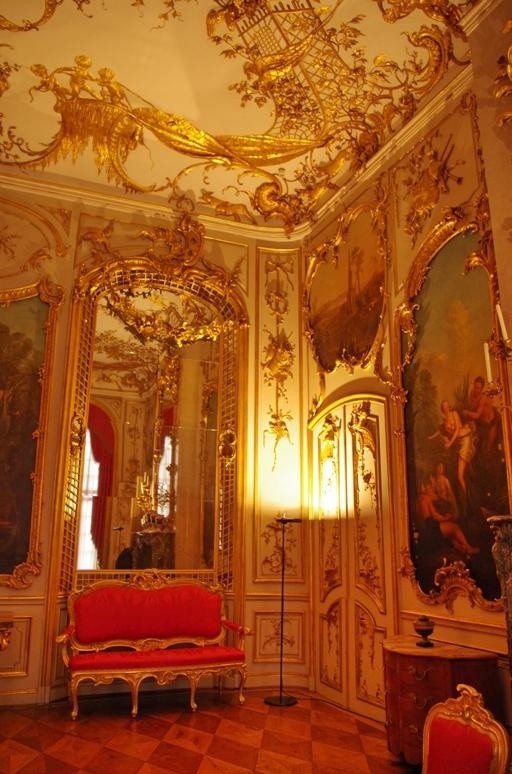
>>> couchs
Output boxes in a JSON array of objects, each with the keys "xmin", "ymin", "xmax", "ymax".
[{"xmin": 56, "ymin": 568, "xmax": 249, "ymax": 720}]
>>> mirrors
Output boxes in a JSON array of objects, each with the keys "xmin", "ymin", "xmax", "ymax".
[{"xmin": 60, "ymin": 258, "xmax": 245, "ymax": 617}]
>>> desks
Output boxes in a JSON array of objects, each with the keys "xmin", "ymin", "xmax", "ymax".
[{"xmin": 382, "ymin": 639, "xmax": 498, "ymax": 766}]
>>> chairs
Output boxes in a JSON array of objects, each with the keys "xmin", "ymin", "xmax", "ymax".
[{"xmin": 423, "ymin": 679, "xmax": 507, "ymax": 772}]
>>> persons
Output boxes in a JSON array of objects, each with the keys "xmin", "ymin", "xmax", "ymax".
[{"xmin": 412, "ymin": 377, "xmax": 503, "ymax": 556}]
